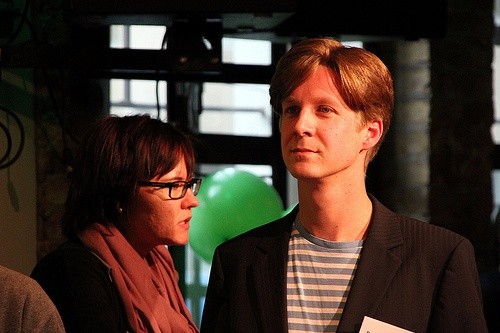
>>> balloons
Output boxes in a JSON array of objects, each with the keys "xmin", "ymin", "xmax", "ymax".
[{"xmin": 187, "ymin": 166, "xmax": 285, "ymax": 263}]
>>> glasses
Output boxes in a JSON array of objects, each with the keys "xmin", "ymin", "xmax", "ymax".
[{"xmin": 138, "ymin": 178, "xmax": 202, "ymax": 200}]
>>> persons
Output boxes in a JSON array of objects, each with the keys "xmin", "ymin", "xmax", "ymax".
[
  {"xmin": 33, "ymin": 111, "xmax": 201, "ymax": 333},
  {"xmin": 0, "ymin": 265, "xmax": 65, "ymax": 333},
  {"xmin": 201, "ymin": 37, "xmax": 487, "ymax": 333}
]
